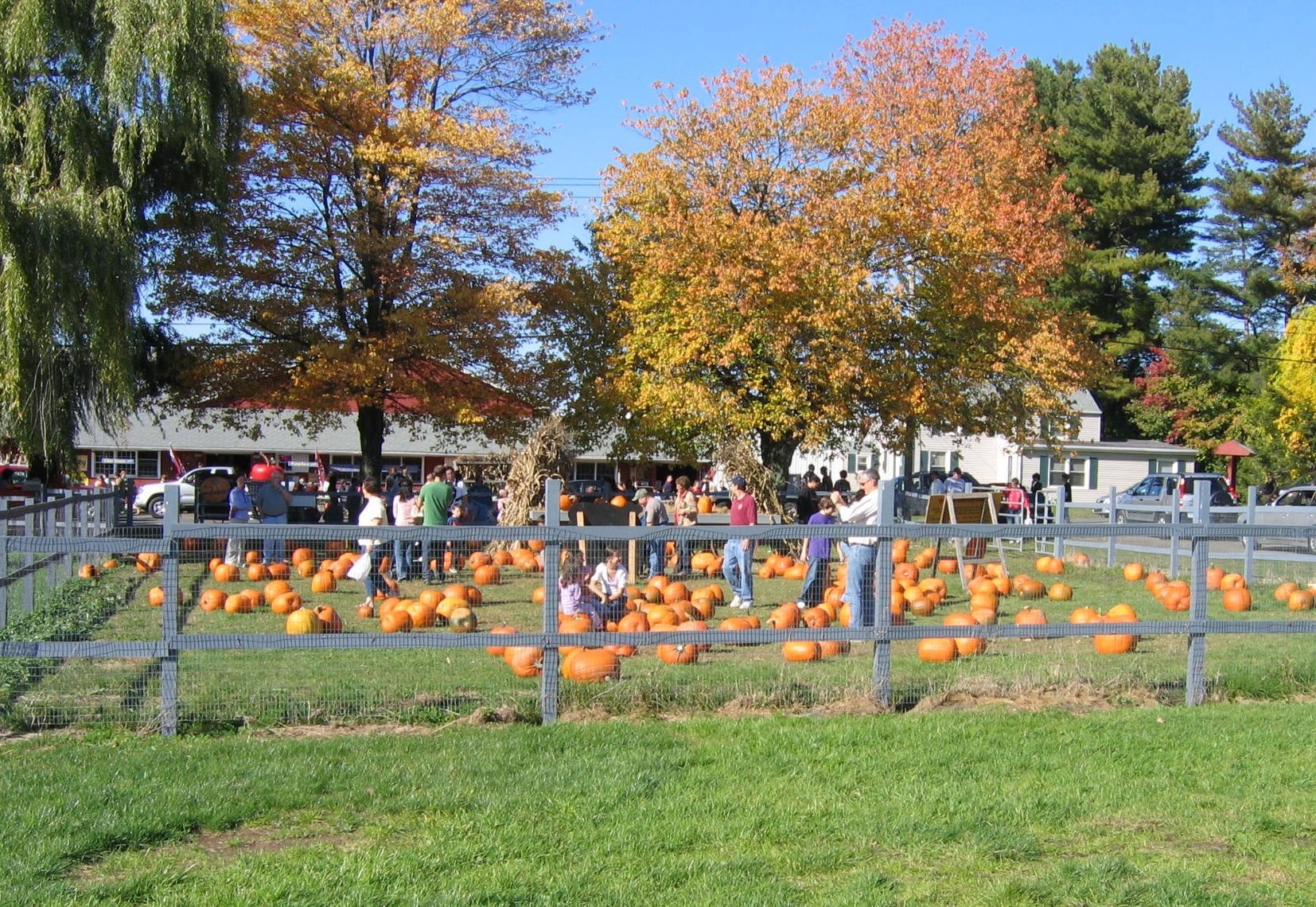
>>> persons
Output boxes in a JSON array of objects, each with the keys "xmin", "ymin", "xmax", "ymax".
[
  {"xmin": 291, "ymin": 464, "xmax": 510, "ymax": 608},
  {"xmin": 95, "ymin": 468, "xmax": 131, "ymax": 513},
  {"xmin": 1003, "ymin": 472, "xmax": 1045, "ymax": 544},
  {"xmin": 928, "ymin": 467, "xmax": 966, "ymax": 496},
  {"xmin": 557, "ymin": 464, "xmax": 880, "ymax": 633},
  {"xmin": 255, "ymin": 468, "xmax": 293, "ymax": 566},
  {"xmin": 224, "ymin": 474, "xmax": 253, "ymax": 567},
  {"xmin": 1061, "ymin": 473, "xmax": 1071, "ymax": 524}
]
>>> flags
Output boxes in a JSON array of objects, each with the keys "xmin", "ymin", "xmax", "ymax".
[
  {"xmin": 169, "ymin": 444, "xmax": 188, "ymax": 477},
  {"xmin": 314, "ymin": 449, "xmax": 326, "ymax": 492}
]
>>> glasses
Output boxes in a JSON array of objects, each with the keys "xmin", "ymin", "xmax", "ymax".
[
  {"xmin": 859, "ymin": 479, "xmax": 877, "ymax": 486},
  {"xmin": 1268, "ymin": 474, "xmax": 1271, "ymax": 475}
]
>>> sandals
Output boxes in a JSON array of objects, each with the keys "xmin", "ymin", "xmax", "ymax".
[
  {"xmin": 383, "ymin": 593, "xmax": 397, "ymax": 599},
  {"xmin": 354, "ymin": 602, "xmax": 374, "ymax": 608}
]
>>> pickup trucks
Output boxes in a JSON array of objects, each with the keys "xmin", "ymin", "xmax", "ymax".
[
  {"xmin": 695, "ymin": 474, "xmax": 857, "ymax": 523},
  {"xmin": 135, "ymin": 466, "xmax": 295, "ymax": 519},
  {"xmin": 541, "ymin": 479, "xmax": 676, "ymax": 504}
]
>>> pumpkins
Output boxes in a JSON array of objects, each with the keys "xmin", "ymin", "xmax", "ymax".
[
  {"xmin": 76, "ymin": 543, "xmax": 856, "ymax": 682},
  {"xmin": 885, "ymin": 538, "xmax": 1316, "ymax": 662},
  {"xmin": 559, "ymin": 495, "xmax": 572, "ymax": 510},
  {"xmin": 610, "ymin": 495, "xmax": 628, "ymax": 508},
  {"xmin": 697, "ymin": 493, "xmax": 712, "ymax": 513}
]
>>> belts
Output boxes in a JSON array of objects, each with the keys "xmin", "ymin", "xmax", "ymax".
[
  {"xmin": 851, "ymin": 542, "xmax": 874, "ymax": 547},
  {"xmin": 266, "ymin": 512, "xmax": 285, "ymax": 517}
]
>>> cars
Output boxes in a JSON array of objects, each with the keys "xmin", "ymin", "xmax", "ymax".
[
  {"xmin": 893, "ymin": 470, "xmax": 1053, "ymax": 525},
  {"xmin": 1236, "ymin": 483, "xmax": 1316, "ymax": 555}
]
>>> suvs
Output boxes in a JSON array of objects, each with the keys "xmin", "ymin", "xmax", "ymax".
[
  {"xmin": 1093, "ymin": 473, "xmax": 1236, "ymax": 540},
  {"xmin": 0, "ymin": 462, "xmax": 125, "ymax": 522}
]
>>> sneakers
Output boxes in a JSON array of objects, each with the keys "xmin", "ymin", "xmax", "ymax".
[
  {"xmin": 730, "ymin": 595, "xmax": 742, "ymax": 608},
  {"xmin": 740, "ymin": 600, "xmax": 754, "ymax": 609}
]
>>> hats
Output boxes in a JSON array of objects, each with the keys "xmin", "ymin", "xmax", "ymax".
[
  {"xmin": 632, "ymin": 488, "xmax": 648, "ymax": 501},
  {"xmin": 728, "ymin": 475, "xmax": 746, "ymax": 489}
]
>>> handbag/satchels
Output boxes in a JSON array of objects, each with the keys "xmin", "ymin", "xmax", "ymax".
[
  {"xmin": 347, "ymin": 548, "xmax": 371, "ymax": 581},
  {"xmin": 412, "ymin": 495, "xmax": 424, "ymax": 524},
  {"xmin": 345, "ymin": 502, "xmax": 350, "ymax": 508},
  {"xmin": 1024, "ymin": 509, "xmax": 1033, "ymax": 525}
]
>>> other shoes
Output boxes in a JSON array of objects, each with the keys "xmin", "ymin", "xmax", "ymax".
[
  {"xmin": 261, "ymin": 560, "xmax": 272, "ymax": 565},
  {"xmin": 273, "ymin": 560, "xmax": 289, "ymax": 565},
  {"xmin": 400, "ymin": 578, "xmax": 407, "ymax": 583},
  {"xmin": 1016, "ymin": 540, "xmax": 1020, "ymax": 543},
  {"xmin": 407, "ymin": 573, "xmax": 416, "ymax": 580},
  {"xmin": 1009, "ymin": 541, "xmax": 1014, "ymax": 544},
  {"xmin": 797, "ymin": 602, "xmax": 806, "ymax": 609}
]
